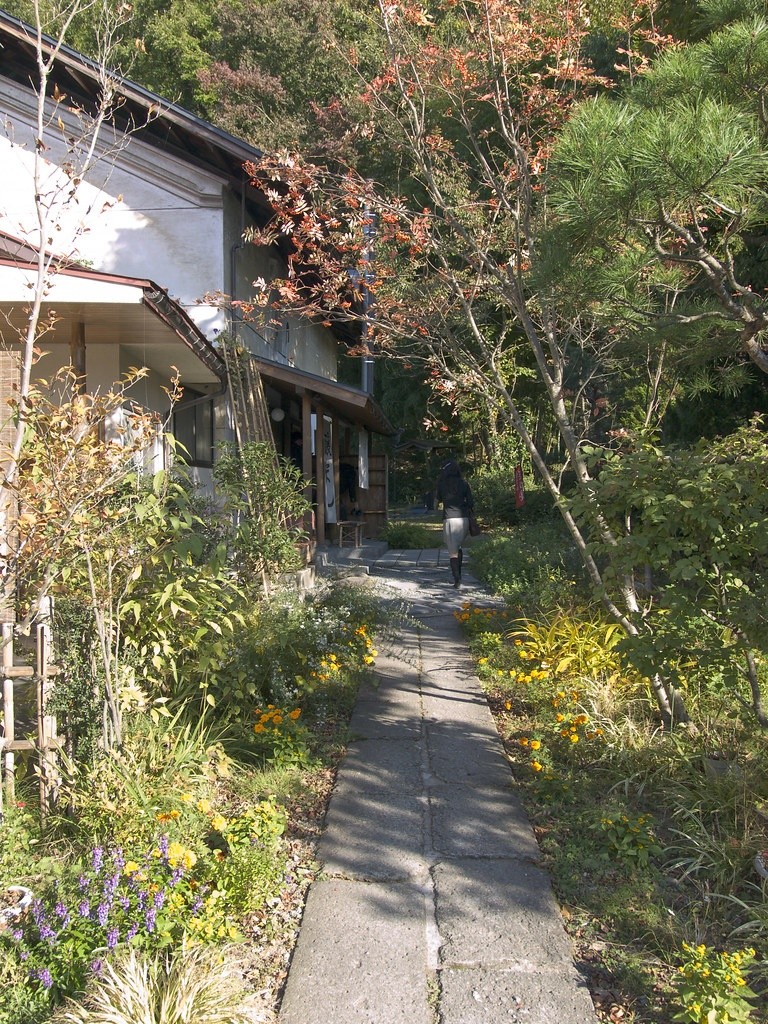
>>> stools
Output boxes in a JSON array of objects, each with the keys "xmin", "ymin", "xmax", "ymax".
[{"xmin": 337, "ymin": 520, "xmax": 367, "ymax": 549}]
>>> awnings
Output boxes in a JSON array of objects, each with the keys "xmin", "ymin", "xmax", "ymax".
[{"xmin": 392, "ymin": 434, "xmax": 456, "ymax": 454}]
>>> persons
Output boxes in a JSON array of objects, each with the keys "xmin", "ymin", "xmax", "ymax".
[{"xmin": 435, "ymin": 454, "xmax": 474, "ymax": 588}]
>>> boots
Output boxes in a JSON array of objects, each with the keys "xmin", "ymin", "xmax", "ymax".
[
  {"xmin": 451, "ymin": 558, "xmax": 461, "ymax": 589},
  {"xmin": 458, "ymin": 549, "xmax": 463, "ymax": 562}
]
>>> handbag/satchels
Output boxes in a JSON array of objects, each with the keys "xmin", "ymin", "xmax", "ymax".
[{"xmin": 467, "ymin": 508, "xmax": 481, "ymax": 537}]
